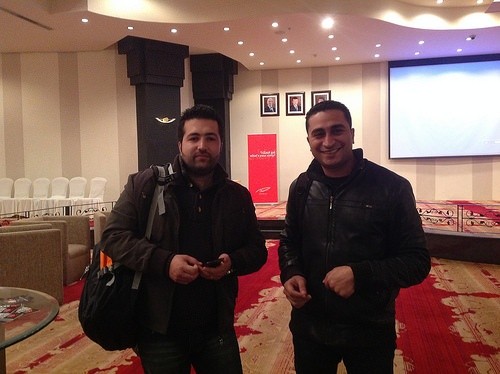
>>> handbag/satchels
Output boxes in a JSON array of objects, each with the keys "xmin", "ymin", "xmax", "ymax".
[{"xmin": 78, "ymin": 264, "xmax": 150, "ymax": 351}]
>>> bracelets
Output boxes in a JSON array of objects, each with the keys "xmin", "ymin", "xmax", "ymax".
[{"xmin": 227, "ymin": 269, "xmax": 232, "ymax": 274}]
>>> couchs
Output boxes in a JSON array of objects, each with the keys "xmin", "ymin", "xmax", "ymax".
[{"xmin": 0, "ymin": 211, "xmax": 111, "ymax": 305}]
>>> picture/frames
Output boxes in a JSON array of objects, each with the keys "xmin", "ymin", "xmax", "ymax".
[
  {"xmin": 311, "ymin": 90, "xmax": 332, "ymax": 109},
  {"xmin": 260, "ymin": 93, "xmax": 279, "ymax": 116},
  {"xmin": 285, "ymin": 92, "xmax": 305, "ymax": 115}
]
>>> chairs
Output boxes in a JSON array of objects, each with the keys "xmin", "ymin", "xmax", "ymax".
[{"xmin": 0, "ymin": 177, "xmax": 106, "ymax": 215}]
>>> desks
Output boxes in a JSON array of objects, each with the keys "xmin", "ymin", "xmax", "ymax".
[{"xmin": 0, "ymin": 285, "xmax": 60, "ymax": 374}]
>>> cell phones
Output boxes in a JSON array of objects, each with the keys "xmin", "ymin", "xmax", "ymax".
[{"xmin": 202, "ymin": 259, "xmax": 221, "ymax": 267}]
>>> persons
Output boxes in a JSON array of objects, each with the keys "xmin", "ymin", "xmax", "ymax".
[
  {"xmin": 291, "ymin": 97, "xmax": 300, "ymax": 111},
  {"xmin": 318, "ymin": 98, "xmax": 324, "ymax": 103},
  {"xmin": 264, "ymin": 98, "xmax": 276, "ymax": 112},
  {"xmin": 99, "ymin": 105, "xmax": 268, "ymax": 374},
  {"xmin": 278, "ymin": 99, "xmax": 431, "ymax": 374}
]
